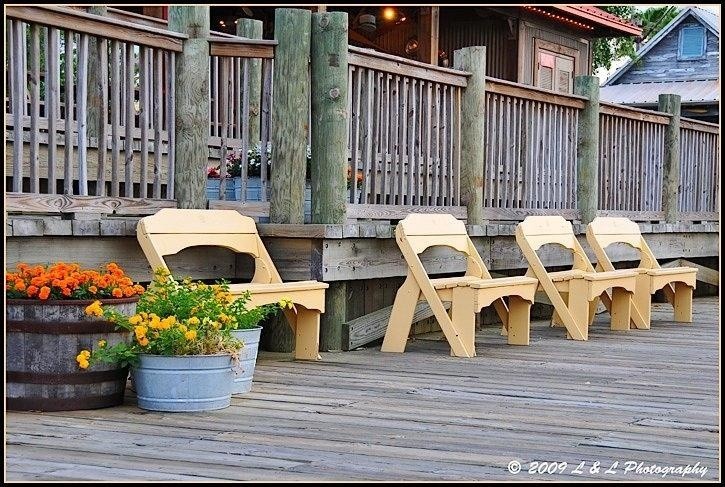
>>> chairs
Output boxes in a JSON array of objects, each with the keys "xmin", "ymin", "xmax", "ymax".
[
  {"xmin": 586, "ymin": 216, "xmax": 699, "ymax": 329},
  {"xmin": 137, "ymin": 208, "xmax": 330, "ymax": 361},
  {"xmin": 381, "ymin": 213, "xmax": 537, "ymax": 357},
  {"xmin": 516, "ymin": 216, "xmax": 640, "ymax": 340}
]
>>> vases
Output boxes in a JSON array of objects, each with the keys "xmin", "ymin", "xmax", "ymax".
[
  {"xmin": 301, "ymin": 179, "xmax": 311, "ymax": 223},
  {"xmin": 6, "ymin": 296, "xmax": 139, "ymax": 411},
  {"xmin": 235, "ymin": 176, "xmax": 270, "ymax": 203},
  {"xmin": 206, "ymin": 177, "xmax": 237, "ymax": 201},
  {"xmin": 131, "ymin": 352, "xmax": 238, "ymax": 412},
  {"xmin": 219, "ymin": 325, "xmax": 263, "ymax": 395}
]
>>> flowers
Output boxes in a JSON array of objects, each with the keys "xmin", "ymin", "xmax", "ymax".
[
  {"xmin": 6, "ymin": 262, "xmax": 145, "ymax": 302},
  {"xmin": 76, "ymin": 291, "xmax": 243, "ymax": 369},
  {"xmin": 215, "ymin": 141, "xmax": 312, "ymax": 171},
  {"xmin": 137, "ymin": 269, "xmax": 292, "ymax": 328}
]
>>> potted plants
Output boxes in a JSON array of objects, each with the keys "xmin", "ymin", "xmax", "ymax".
[{"xmin": 346, "ymin": 165, "xmax": 362, "ymax": 222}]
